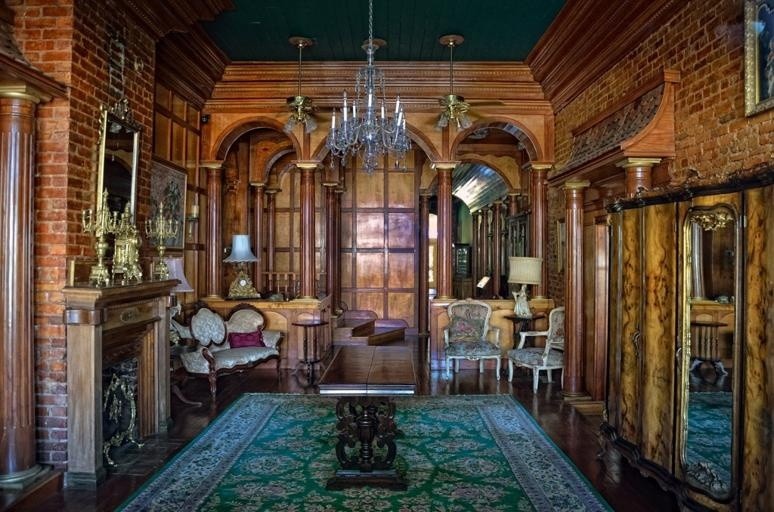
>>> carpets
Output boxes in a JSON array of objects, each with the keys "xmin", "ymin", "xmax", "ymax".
[{"xmin": 113, "ymin": 394, "xmax": 611, "ymax": 512}]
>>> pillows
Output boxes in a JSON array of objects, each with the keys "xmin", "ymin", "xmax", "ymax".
[{"xmin": 228, "ymin": 330, "xmax": 266, "ymax": 348}]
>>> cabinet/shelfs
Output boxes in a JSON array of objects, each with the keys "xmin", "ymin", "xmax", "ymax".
[{"xmin": 606, "ymin": 151, "xmax": 774, "ymax": 512}]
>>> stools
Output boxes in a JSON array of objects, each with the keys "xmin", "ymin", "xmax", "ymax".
[{"xmin": 292, "ymin": 319, "xmax": 327, "ymax": 388}]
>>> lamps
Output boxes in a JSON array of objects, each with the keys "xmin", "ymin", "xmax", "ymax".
[
  {"xmin": 166, "ymin": 259, "xmax": 193, "ymax": 317},
  {"xmin": 284, "ymin": 36, "xmax": 317, "ymax": 135},
  {"xmin": 223, "ymin": 235, "xmax": 261, "ymax": 300},
  {"xmin": 508, "ymin": 257, "xmax": 542, "ymax": 317},
  {"xmin": 438, "ymin": 34, "xmax": 474, "ymax": 130},
  {"xmin": 326, "ymin": 0, "xmax": 413, "ymax": 176}
]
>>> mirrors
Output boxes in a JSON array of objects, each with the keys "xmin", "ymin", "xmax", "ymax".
[
  {"xmin": 679, "ymin": 206, "xmax": 737, "ymax": 504},
  {"xmin": 96, "ymin": 100, "xmax": 142, "ymax": 238}
]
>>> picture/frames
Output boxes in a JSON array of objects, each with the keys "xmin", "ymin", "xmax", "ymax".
[
  {"xmin": 743, "ymin": 2, "xmax": 774, "ymax": 116},
  {"xmin": 150, "ymin": 155, "xmax": 188, "ymax": 250}
]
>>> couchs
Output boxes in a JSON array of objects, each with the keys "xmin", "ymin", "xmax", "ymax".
[
  {"xmin": 166, "ymin": 300, "xmax": 284, "ymax": 419},
  {"xmin": 443, "ymin": 298, "xmax": 503, "ymax": 381},
  {"xmin": 508, "ymin": 307, "xmax": 566, "ymax": 393}
]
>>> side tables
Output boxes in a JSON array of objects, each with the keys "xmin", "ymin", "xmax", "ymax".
[{"xmin": 505, "ymin": 316, "xmax": 544, "ymax": 376}]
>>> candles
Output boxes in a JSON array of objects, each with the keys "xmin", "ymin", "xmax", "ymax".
[{"xmin": 191, "ymin": 204, "xmax": 198, "ymax": 215}]
